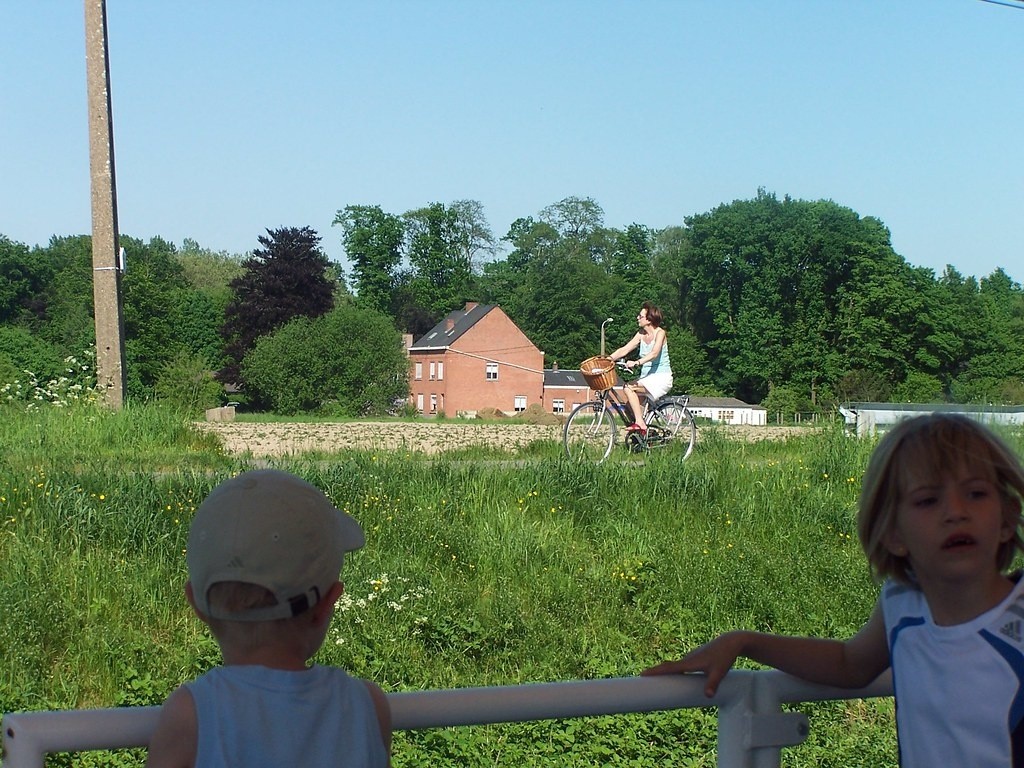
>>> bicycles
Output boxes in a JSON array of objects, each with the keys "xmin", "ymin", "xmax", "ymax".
[{"xmin": 563, "ymin": 353, "xmax": 699, "ymax": 468}]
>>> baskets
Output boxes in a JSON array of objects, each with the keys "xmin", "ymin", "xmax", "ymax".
[{"xmin": 581, "ymin": 354, "xmax": 618, "ymax": 390}]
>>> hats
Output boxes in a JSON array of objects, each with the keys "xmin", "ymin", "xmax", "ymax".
[{"xmin": 187, "ymin": 470, "xmax": 366, "ymax": 624}]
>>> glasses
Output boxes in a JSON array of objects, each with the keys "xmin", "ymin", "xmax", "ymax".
[{"xmin": 640, "ymin": 315, "xmax": 646, "ymax": 319}]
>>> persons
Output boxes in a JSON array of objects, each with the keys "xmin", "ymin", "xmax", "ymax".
[
  {"xmin": 145, "ymin": 469, "xmax": 392, "ymax": 768},
  {"xmin": 605, "ymin": 303, "xmax": 674, "ymax": 431},
  {"xmin": 639, "ymin": 412, "xmax": 1024, "ymax": 768}
]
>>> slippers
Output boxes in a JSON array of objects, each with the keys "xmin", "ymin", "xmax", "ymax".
[{"xmin": 624, "ymin": 424, "xmax": 647, "ymax": 435}]
